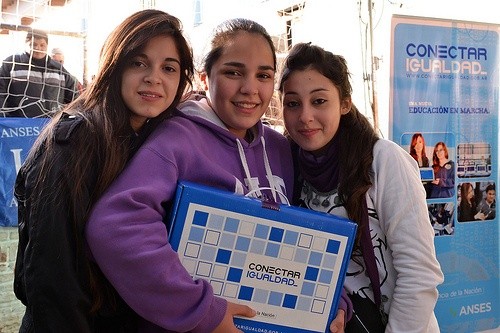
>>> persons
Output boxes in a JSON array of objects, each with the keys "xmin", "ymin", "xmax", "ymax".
[
  {"xmin": 408, "ymin": 132, "xmax": 496, "ymax": 234},
  {"xmin": 275, "ymin": 40, "xmax": 445, "ymax": 333},
  {"xmin": 0, "ymin": 28, "xmax": 96, "ymax": 118},
  {"xmin": 12, "ymin": 10, "xmax": 197, "ymax": 333},
  {"xmin": 83, "ymin": 18, "xmax": 354, "ymax": 333}
]
